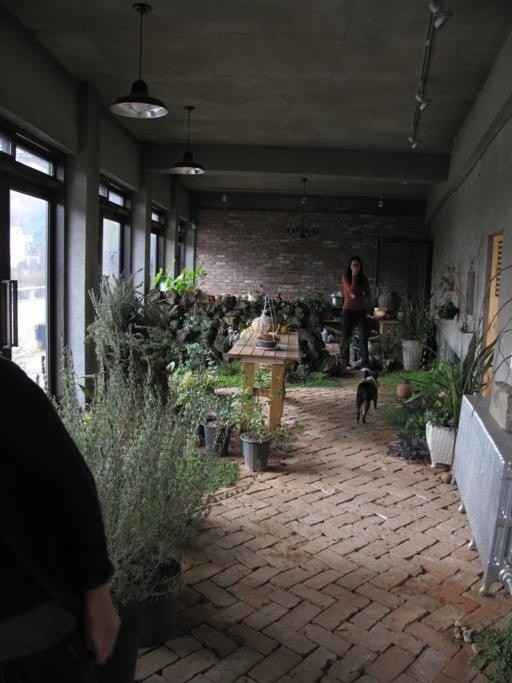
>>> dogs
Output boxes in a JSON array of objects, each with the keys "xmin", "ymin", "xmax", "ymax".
[{"xmin": 356, "ymin": 369, "xmax": 379, "ymax": 423}]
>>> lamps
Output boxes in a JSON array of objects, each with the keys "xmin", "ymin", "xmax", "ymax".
[
  {"xmin": 285, "ymin": 177, "xmax": 321, "ymax": 242},
  {"xmin": 407, "ymin": 1, "xmax": 452, "ymax": 151},
  {"xmin": 108, "ymin": 1, "xmax": 170, "ymax": 120},
  {"xmin": 171, "ymin": 105, "xmax": 206, "ymax": 177}
]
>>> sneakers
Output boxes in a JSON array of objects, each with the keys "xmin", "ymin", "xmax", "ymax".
[{"xmin": 347, "ymin": 365, "xmax": 370, "ymax": 372}]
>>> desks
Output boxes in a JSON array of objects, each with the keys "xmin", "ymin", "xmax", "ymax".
[{"xmin": 226, "ymin": 327, "xmax": 300, "ymax": 432}]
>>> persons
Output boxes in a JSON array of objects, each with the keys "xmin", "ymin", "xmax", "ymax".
[
  {"xmin": 340, "ymin": 254, "xmax": 372, "ymax": 370},
  {"xmin": 1, "ymin": 356, "xmax": 121, "ymax": 682}
]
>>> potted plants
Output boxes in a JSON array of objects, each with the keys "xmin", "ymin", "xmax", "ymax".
[
  {"xmin": 398, "ymin": 264, "xmax": 512, "ymax": 469},
  {"xmin": 35, "ymin": 331, "xmax": 305, "ymax": 683}
]
